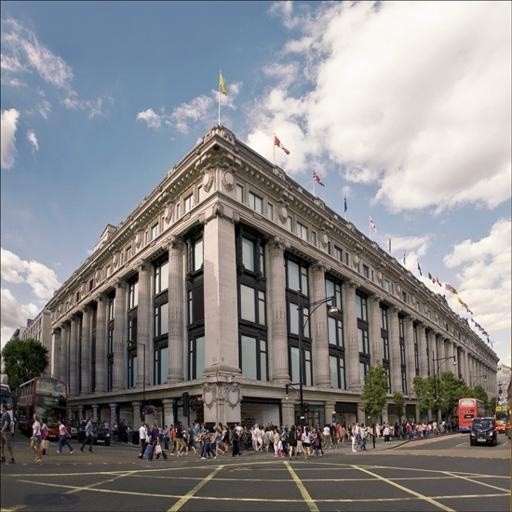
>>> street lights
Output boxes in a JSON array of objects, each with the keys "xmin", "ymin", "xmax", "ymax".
[
  {"xmin": 285, "ymin": 382, "xmax": 307, "ymax": 435},
  {"xmin": 295, "ymin": 289, "xmax": 341, "ymax": 426},
  {"xmin": 430, "ymin": 350, "xmax": 457, "ymax": 425},
  {"xmin": 126, "ymin": 339, "xmax": 147, "ymax": 415}
]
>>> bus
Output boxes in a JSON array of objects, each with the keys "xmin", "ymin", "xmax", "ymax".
[
  {"xmin": 457, "ymin": 397, "xmax": 486, "ymax": 433},
  {"xmin": 15, "ymin": 375, "xmax": 71, "ymax": 440},
  {"xmin": 0, "ymin": 383, "xmax": 17, "ymax": 435},
  {"xmin": 495, "ymin": 405, "xmax": 511, "ymax": 434}
]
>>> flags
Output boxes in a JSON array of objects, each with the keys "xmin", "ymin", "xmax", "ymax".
[
  {"xmin": 418, "ymin": 263, "xmax": 422, "ymax": 275},
  {"xmin": 219, "ymin": 74, "xmax": 228, "ymax": 96},
  {"xmin": 369, "ymin": 218, "xmax": 377, "ymax": 233},
  {"xmin": 344, "ymin": 197, "xmax": 347, "ymax": 211},
  {"xmin": 274, "ymin": 132, "xmax": 290, "ymax": 155},
  {"xmin": 313, "ymin": 171, "xmax": 326, "ymax": 187},
  {"xmin": 429, "ymin": 273, "xmax": 489, "ymax": 343}
]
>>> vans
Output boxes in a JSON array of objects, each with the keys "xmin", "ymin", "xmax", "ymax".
[{"xmin": 468, "ymin": 416, "xmax": 497, "ymax": 446}]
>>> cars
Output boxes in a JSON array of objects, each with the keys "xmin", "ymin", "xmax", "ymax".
[{"xmin": 77, "ymin": 419, "xmax": 112, "ymax": 446}]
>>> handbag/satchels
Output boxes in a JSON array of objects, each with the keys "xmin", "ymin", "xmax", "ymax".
[
  {"xmin": 40, "ymin": 424, "xmax": 48, "ymax": 439},
  {"xmin": 153, "ymin": 444, "xmax": 163, "ymax": 456}
]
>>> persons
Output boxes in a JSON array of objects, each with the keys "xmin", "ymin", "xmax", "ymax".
[
  {"xmin": 30, "ymin": 414, "xmax": 50, "ymax": 463},
  {"xmin": 0, "ymin": 403, "xmax": 18, "ymax": 464},
  {"xmin": 80, "ymin": 417, "xmax": 97, "ymax": 451},
  {"xmin": 57, "ymin": 419, "xmax": 75, "ymax": 454}
]
